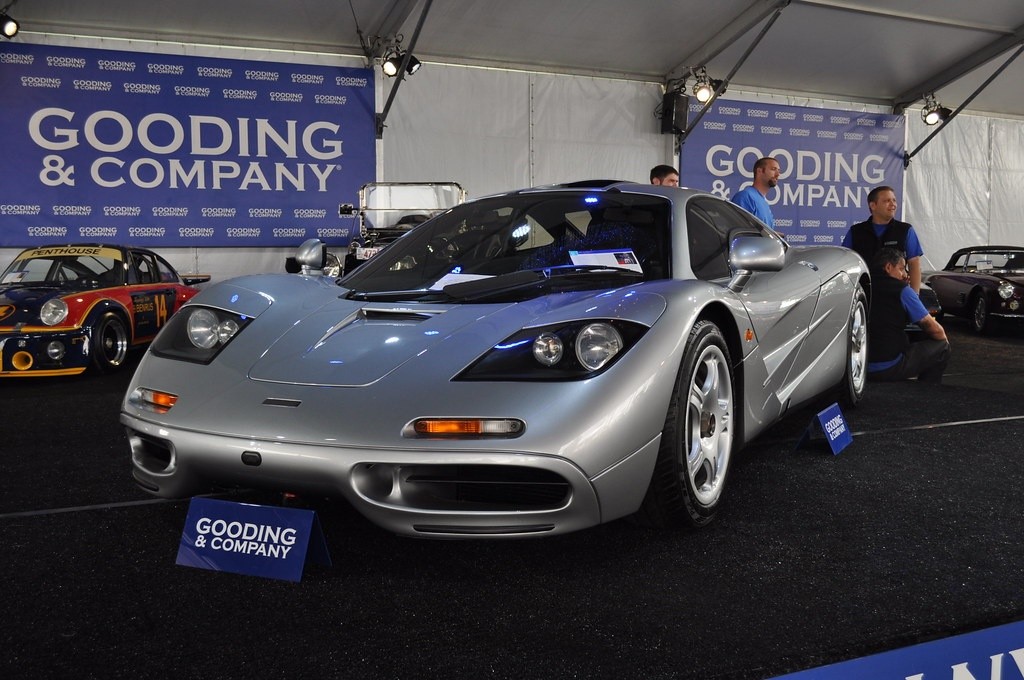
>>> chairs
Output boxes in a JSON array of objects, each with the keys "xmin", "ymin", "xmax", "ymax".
[
  {"xmin": 584, "ymin": 218, "xmax": 667, "ymax": 267},
  {"xmin": 1004, "ymin": 259, "xmax": 1019, "ymax": 268}
]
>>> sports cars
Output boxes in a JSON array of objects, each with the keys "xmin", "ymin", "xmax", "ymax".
[
  {"xmin": 114, "ymin": 179, "xmax": 875, "ymax": 540},
  {"xmin": 921, "ymin": 245, "xmax": 1023, "ymax": 330},
  {"xmin": 0, "ymin": 246, "xmax": 211, "ymax": 380}
]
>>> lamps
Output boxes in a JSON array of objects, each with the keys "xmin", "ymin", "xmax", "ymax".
[
  {"xmin": 921, "ymin": 96, "xmax": 952, "ymax": 125},
  {"xmin": 381, "ymin": 43, "xmax": 421, "ymax": 76},
  {"xmin": 0, "ymin": 10, "xmax": 20, "ymax": 39},
  {"xmin": 688, "ymin": 66, "xmax": 727, "ymax": 103}
]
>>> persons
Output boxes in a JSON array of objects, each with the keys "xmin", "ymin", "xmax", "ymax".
[
  {"xmin": 842, "ymin": 187, "xmax": 924, "ymax": 296},
  {"xmin": 865, "ymin": 247, "xmax": 951, "ymax": 383},
  {"xmin": 732, "ymin": 157, "xmax": 785, "ymax": 238},
  {"xmin": 650, "ymin": 165, "xmax": 680, "ymax": 187}
]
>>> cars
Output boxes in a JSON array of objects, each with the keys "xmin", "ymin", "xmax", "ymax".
[{"xmin": 320, "ymin": 183, "xmax": 530, "ymax": 277}]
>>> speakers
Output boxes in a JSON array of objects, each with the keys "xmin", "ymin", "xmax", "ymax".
[{"xmin": 660, "ymin": 93, "xmax": 688, "ymax": 135}]
